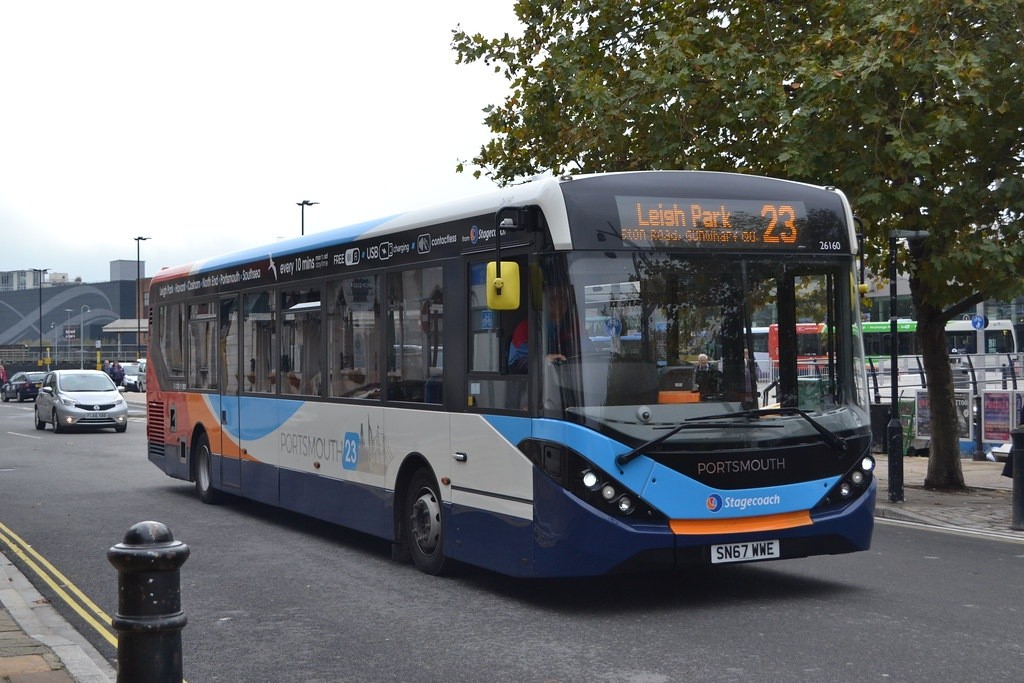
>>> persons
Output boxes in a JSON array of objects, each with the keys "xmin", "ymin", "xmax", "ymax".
[
  {"xmin": 744, "ymin": 349, "xmax": 760, "ymax": 383},
  {"xmin": 692, "ymin": 353, "xmax": 718, "ymax": 388},
  {"xmin": 103, "ymin": 359, "xmax": 125, "ymax": 387},
  {"xmin": 508, "ymin": 276, "xmax": 598, "ymax": 372}
]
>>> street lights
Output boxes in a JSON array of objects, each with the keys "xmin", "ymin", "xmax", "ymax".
[
  {"xmin": 65, "ymin": 308, "xmax": 74, "ymax": 357},
  {"xmin": 32, "ymin": 268, "xmax": 52, "ymax": 371},
  {"xmin": 133, "ymin": 236, "xmax": 152, "ymax": 359},
  {"xmin": 295, "ymin": 200, "xmax": 320, "ymax": 235},
  {"xmin": 50, "ymin": 322, "xmax": 58, "ymax": 366},
  {"xmin": 79, "ymin": 305, "xmax": 94, "ymax": 369},
  {"xmin": 886, "ymin": 227, "xmax": 933, "ymax": 503}
]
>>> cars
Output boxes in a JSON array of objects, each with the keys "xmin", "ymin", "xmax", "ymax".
[
  {"xmin": 108, "ymin": 357, "xmax": 147, "ymax": 393},
  {"xmin": 34, "ymin": 369, "xmax": 128, "ymax": 434},
  {"xmin": 0, "ymin": 371, "xmax": 50, "ymax": 402}
]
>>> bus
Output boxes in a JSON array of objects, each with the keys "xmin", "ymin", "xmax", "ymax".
[
  {"xmin": 143, "ymin": 169, "xmax": 878, "ymax": 578},
  {"xmin": 589, "ymin": 316, "xmax": 1017, "ymax": 411}
]
